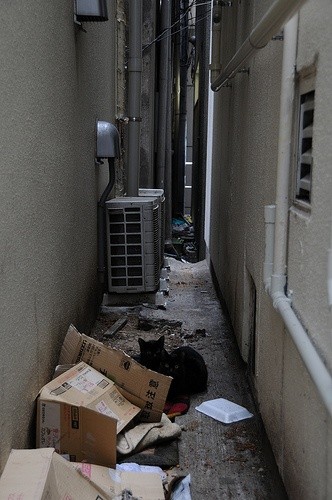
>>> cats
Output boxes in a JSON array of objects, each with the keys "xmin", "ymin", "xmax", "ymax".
[{"xmin": 130, "ymin": 335, "xmax": 209, "ymax": 396}]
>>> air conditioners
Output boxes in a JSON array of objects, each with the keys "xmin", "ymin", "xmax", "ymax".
[
  {"xmin": 101, "ymin": 197, "xmax": 162, "ymax": 295},
  {"xmin": 125, "ymin": 188, "xmax": 165, "ymax": 269}
]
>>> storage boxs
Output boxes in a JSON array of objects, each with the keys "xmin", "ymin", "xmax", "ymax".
[{"xmin": 0, "ymin": 322, "xmax": 194, "ymax": 500}]
